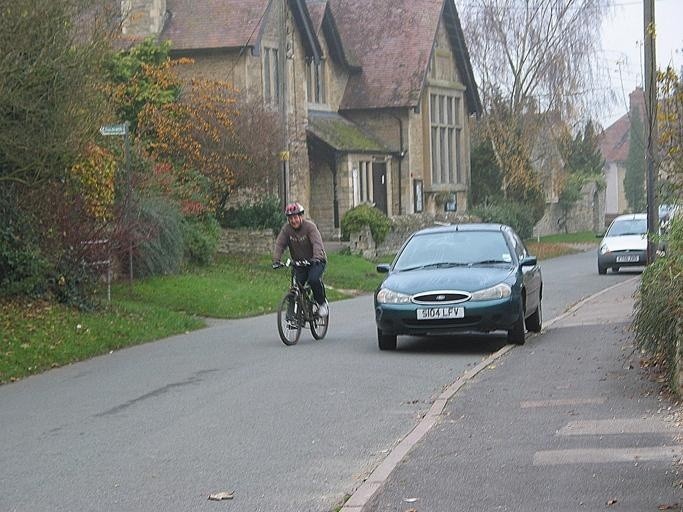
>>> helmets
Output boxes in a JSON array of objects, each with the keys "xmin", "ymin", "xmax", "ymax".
[{"xmin": 285, "ymin": 202, "xmax": 304, "ymax": 216}]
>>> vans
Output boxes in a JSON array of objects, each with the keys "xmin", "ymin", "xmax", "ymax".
[{"xmin": 594, "ymin": 212, "xmax": 665, "ymax": 276}]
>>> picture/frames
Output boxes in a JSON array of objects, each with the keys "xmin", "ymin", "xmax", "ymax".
[
  {"xmin": 414, "ymin": 178, "xmax": 423, "ymax": 213},
  {"xmin": 445, "ymin": 192, "xmax": 457, "ymax": 212}
]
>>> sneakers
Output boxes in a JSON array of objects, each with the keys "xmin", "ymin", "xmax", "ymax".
[{"xmin": 318, "ymin": 301, "xmax": 329, "ymax": 317}]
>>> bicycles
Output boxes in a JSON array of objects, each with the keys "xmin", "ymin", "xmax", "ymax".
[{"xmin": 272, "ymin": 259, "xmax": 329, "ymax": 347}]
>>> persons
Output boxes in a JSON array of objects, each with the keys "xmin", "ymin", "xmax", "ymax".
[{"xmin": 271, "ymin": 202, "xmax": 329, "ymax": 331}]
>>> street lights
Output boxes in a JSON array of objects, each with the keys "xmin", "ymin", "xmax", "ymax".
[{"xmin": 280, "ymin": 150, "xmax": 289, "ymax": 216}]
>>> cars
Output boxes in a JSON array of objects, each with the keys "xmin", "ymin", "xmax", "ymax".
[
  {"xmin": 373, "ymin": 223, "xmax": 543, "ymax": 354},
  {"xmin": 642, "ymin": 205, "xmax": 679, "ymax": 220}
]
